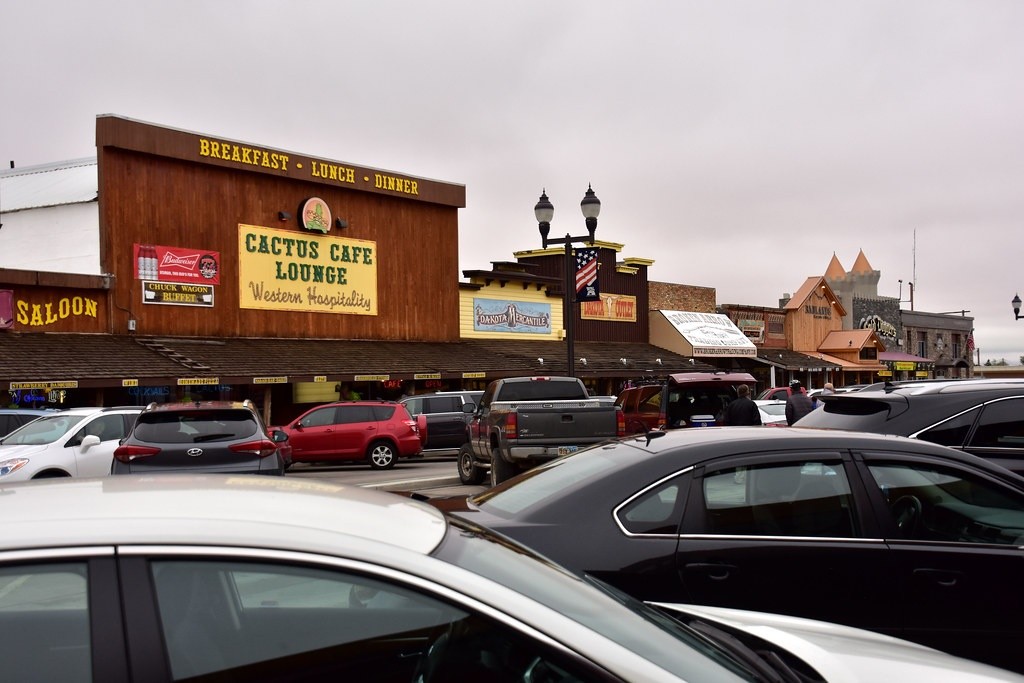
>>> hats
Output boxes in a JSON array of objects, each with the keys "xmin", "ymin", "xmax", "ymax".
[{"xmin": 824, "ymin": 383, "xmax": 834, "ymax": 390}]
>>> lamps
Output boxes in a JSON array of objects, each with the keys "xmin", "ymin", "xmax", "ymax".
[
  {"xmin": 579, "ymin": 357, "xmax": 588, "ymax": 365},
  {"xmin": 689, "ymin": 359, "xmax": 695, "ymax": 366},
  {"xmin": 620, "ymin": 356, "xmax": 626, "ymax": 364},
  {"xmin": 655, "ymin": 358, "xmax": 662, "ymax": 366},
  {"xmin": 536, "ymin": 353, "xmax": 544, "ymax": 365}
]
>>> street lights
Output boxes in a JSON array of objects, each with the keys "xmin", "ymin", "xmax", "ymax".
[{"xmin": 532, "ymin": 182, "xmax": 601, "ymax": 378}]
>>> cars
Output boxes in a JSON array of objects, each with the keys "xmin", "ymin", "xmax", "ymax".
[
  {"xmin": 428, "ymin": 426, "xmax": 1023, "ymax": 674},
  {"xmin": 0, "ymin": 474, "xmax": 1024, "ymax": 683}
]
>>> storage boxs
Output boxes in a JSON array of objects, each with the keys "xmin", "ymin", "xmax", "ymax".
[{"xmin": 690, "ymin": 414, "xmax": 716, "ymax": 427}]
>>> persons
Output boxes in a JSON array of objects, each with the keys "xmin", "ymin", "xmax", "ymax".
[
  {"xmin": 723, "ymin": 384, "xmax": 762, "ymax": 427},
  {"xmin": 816, "ymin": 383, "xmax": 835, "ymax": 408},
  {"xmin": 784, "ymin": 380, "xmax": 814, "ymax": 427}
]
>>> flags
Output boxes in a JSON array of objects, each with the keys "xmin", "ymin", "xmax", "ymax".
[{"xmin": 966, "ymin": 329, "xmax": 975, "ymax": 351}]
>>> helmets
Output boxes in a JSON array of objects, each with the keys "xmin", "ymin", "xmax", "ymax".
[{"xmin": 789, "ymin": 380, "xmax": 800, "ymax": 388}]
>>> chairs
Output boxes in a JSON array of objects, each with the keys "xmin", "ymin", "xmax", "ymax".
[
  {"xmin": 543, "ymin": 382, "xmax": 566, "ymax": 397},
  {"xmin": 154, "ymin": 564, "xmax": 230, "ymax": 683},
  {"xmin": 774, "ymin": 482, "xmax": 852, "ymax": 537},
  {"xmin": 156, "ymin": 416, "xmax": 192, "ymax": 442},
  {"xmin": 752, "ymin": 464, "xmax": 801, "ymax": 525}
]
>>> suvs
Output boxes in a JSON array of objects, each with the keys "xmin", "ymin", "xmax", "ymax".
[
  {"xmin": 611, "ymin": 367, "xmax": 758, "ymax": 438},
  {"xmin": 0, "ymin": 398, "xmax": 289, "ymax": 477},
  {"xmin": 266, "ymin": 398, "xmax": 429, "ymax": 470},
  {"xmin": 395, "ymin": 390, "xmax": 486, "ymax": 453},
  {"xmin": 754, "ymin": 378, "xmax": 1024, "ymax": 479}
]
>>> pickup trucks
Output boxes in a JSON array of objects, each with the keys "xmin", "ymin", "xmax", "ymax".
[{"xmin": 456, "ymin": 375, "xmax": 628, "ymax": 487}]
